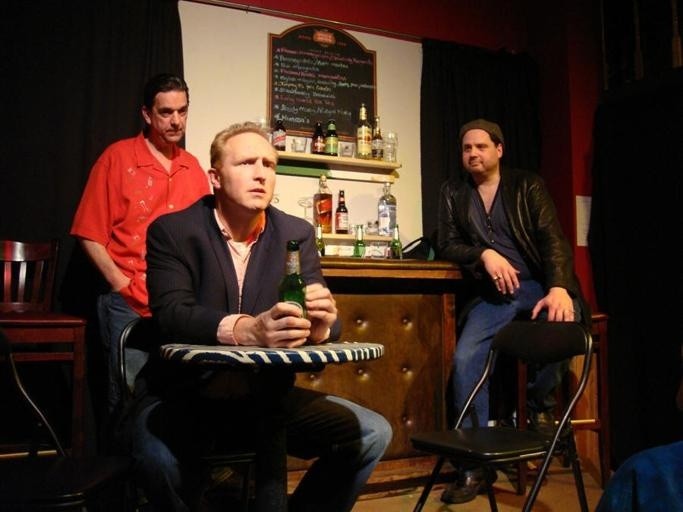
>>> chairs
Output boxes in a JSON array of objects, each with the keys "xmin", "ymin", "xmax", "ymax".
[
  {"xmin": 413, "ymin": 318, "xmax": 593, "ymax": 512},
  {"xmin": 509, "ymin": 311, "xmax": 610, "ymax": 490},
  {"xmin": 116, "ymin": 316, "xmax": 320, "ymax": 512},
  {"xmin": 0, "ymin": 238, "xmax": 89, "ymax": 492}
]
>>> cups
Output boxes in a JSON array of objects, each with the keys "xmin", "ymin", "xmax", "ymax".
[
  {"xmin": 292, "ymin": 138, "xmax": 307, "ymax": 151},
  {"xmin": 382, "ymin": 131, "xmax": 398, "ymax": 163},
  {"xmin": 370, "ymin": 240, "xmax": 388, "ymax": 260},
  {"xmin": 368, "ymin": 220, "xmax": 378, "ymax": 234},
  {"xmin": 339, "ymin": 141, "xmax": 356, "ymax": 159}
]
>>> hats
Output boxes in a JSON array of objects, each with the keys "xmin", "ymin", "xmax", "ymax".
[{"xmin": 459, "ymin": 118, "xmax": 502, "ymax": 142}]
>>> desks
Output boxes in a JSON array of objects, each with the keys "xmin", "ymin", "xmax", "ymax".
[{"xmin": 158, "ymin": 341, "xmax": 385, "ymax": 511}]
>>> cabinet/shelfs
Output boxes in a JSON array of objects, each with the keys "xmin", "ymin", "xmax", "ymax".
[{"xmin": 271, "ymin": 149, "xmax": 402, "ymax": 242}]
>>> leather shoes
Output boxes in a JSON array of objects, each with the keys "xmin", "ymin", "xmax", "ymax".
[{"xmin": 505, "ymin": 393, "xmax": 532, "ymax": 430}]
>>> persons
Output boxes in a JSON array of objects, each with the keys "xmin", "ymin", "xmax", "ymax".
[
  {"xmin": 126, "ymin": 121, "xmax": 394, "ymax": 512},
  {"xmin": 434, "ymin": 117, "xmax": 593, "ymax": 503},
  {"xmin": 595, "ymin": 439, "xmax": 683, "ymax": 511},
  {"xmin": 69, "ymin": 71, "xmax": 211, "ymax": 512}
]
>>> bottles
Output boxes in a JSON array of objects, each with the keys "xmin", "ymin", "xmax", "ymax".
[
  {"xmin": 311, "ymin": 118, "xmax": 338, "ymax": 156},
  {"xmin": 354, "ymin": 224, "xmax": 366, "ymax": 256},
  {"xmin": 314, "ymin": 172, "xmax": 333, "ymax": 235},
  {"xmin": 377, "ymin": 180, "xmax": 398, "ymax": 237},
  {"xmin": 277, "ymin": 238, "xmax": 306, "ymax": 318},
  {"xmin": 391, "ymin": 224, "xmax": 403, "ymax": 260},
  {"xmin": 315, "ymin": 224, "xmax": 325, "ymax": 257},
  {"xmin": 271, "ymin": 118, "xmax": 286, "ymax": 150},
  {"xmin": 335, "ymin": 189, "xmax": 348, "ymax": 232},
  {"xmin": 355, "ymin": 102, "xmax": 386, "ymax": 160}
]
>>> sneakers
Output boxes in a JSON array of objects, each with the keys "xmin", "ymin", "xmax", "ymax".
[{"xmin": 439, "ymin": 468, "xmax": 497, "ymax": 503}]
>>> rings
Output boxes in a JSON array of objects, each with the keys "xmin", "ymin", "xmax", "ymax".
[
  {"xmin": 492, "ymin": 276, "xmax": 497, "ymax": 282},
  {"xmin": 497, "ymin": 272, "xmax": 503, "ymax": 279}
]
